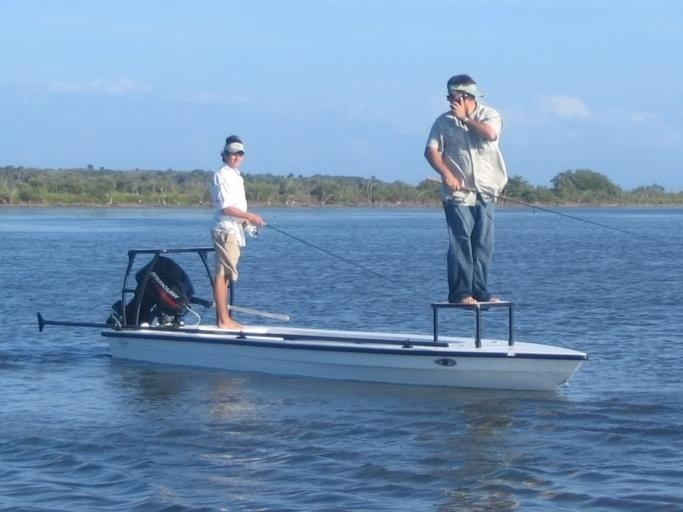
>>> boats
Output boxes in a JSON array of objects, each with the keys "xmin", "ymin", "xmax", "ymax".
[{"xmin": 99, "ymin": 244, "xmax": 588, "ymax": 390}]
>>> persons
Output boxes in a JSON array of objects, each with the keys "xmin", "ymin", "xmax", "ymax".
[
  {"xmin": 206, "ymin": 135, "xmax": 265, "ymax": 332},
  {"xmin": 423, "ymin": 75, "xmax": 510, "ymax": 305}
]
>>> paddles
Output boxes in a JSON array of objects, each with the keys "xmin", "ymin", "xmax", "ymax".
[{"xmin": 35, "ymin": 312, "xmax": 448, "ymax": 347}]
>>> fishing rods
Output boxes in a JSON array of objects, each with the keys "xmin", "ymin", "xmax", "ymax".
[
  {"xmin": 262, "ymin": 221, "xmax": 404, "ymax": 286},
  {"xmin": 424, "ymin": 177, "xmax": 658, "ymax": 243}
]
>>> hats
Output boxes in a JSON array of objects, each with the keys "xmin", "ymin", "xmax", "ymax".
[
  {"xmin": 224, "ymin": 142, "xmax": 245, "ymax": 154},
  {"xmin": 448, "ymin": 83, "xmax": 484, "ymax": 97}
]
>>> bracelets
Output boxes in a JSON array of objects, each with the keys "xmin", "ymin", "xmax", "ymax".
[{"xmin": 462, "ymin": 116, "xmax": 468, "ymax": 123}]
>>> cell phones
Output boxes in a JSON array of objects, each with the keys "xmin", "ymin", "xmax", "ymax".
[{"xmin": 456, "ymin": 92, "xmax": 470, "ymax": 104}]
